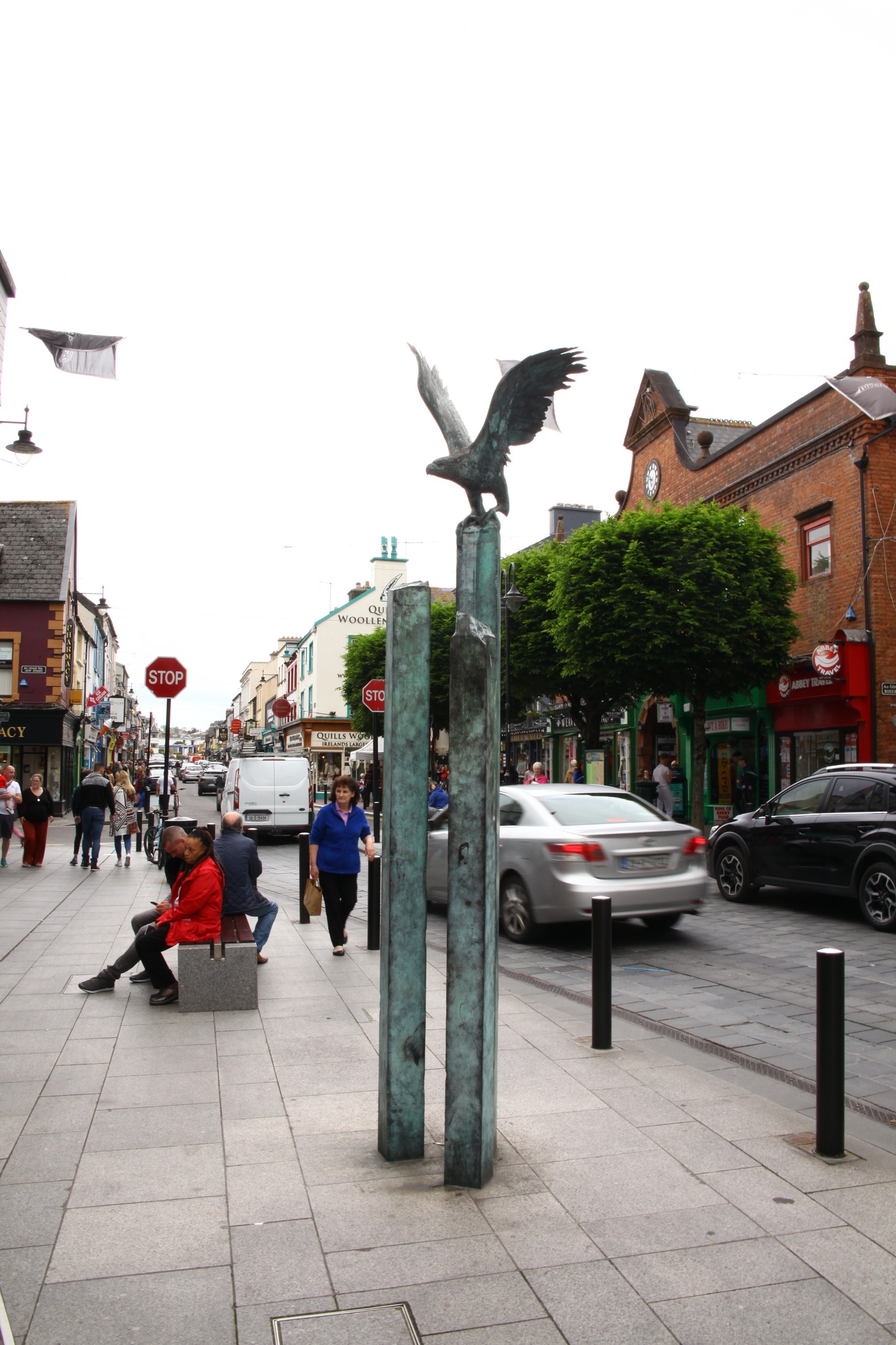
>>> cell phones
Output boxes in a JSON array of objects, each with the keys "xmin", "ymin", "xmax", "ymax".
[
  {"xmin": 144, "ymin": 925, "xmax": 156, "ymax": 935},
  {"xmin": 151, "ymin": 901, "xmax": 158, "ymax": 906}
]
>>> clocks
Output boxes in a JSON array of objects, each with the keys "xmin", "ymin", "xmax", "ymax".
[{"xmin": 644, "ymin": 459, "xmax": 660, "ymax": 501}]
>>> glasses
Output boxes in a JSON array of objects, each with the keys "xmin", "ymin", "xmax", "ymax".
[
  {"xmin": 671, "ymin": 764, "xmax": 678, "ymax": 766},
  {"xmin": 32, "ymin": 780, "xmax": 40, "ymax": 782}
]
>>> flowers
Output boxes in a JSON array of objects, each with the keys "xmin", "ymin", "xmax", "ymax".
[{"xmin": 527, "ymin": 708, "xmax": 541, "ymax": 717}]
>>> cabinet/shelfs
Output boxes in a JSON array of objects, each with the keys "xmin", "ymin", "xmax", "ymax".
[{"xmin": 620, "ymin": 737, "xmax": 630, "ymax": 792}]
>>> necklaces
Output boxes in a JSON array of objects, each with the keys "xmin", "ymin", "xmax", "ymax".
[{"xmin": 32, "ymin": 788, "xmax": 41, "ymax": 802}]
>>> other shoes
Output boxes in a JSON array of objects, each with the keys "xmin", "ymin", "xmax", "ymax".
[
  {"xmin": 70, "ymin": 856, "xmax": 79, "ymax": 865},
  {"xmin": 91, "ymin": 865, "xmax": 100, "ymax": 871},
  {"xmin": 257, "ymin": 953, "xmax": 268, "ymax": 964},
  {"xmin": 343, "ymin": 937, "xmax": 348, "ymax": 944},
  {"xmin": 20, "ymin": 837, "xmax": 24, "ymax": 848},
  {"xmin": 81, "ymin": 858, "xmax": 84, "ymax": 866},
  {"xmin": 83, "ymin": 863, "xmax": 91, "ymax": 868},
  {"xmin": 137, "ymin": 809, "xmax": 141, "ymax": 811},
  {"xmin": 35, "ymin": 863, "xmax": 41, "ymax": 866},
  {"xmin": 115, "ymin": 860, "xmax": 123, "ymax": 866},
  {"xmin": 22, "ymin": 862, "xmax": 29, "ymax": 867},
  {"xmin": 333, "ymin": 948, "xmax": 344, "ymax": 956},
  {"xmin": 124, "ymin": 854, "xmax": 130, "ymax": 866},
  {"xmin": 150, "ymin": 987, "xmax": 179, "ymax": 1005}
]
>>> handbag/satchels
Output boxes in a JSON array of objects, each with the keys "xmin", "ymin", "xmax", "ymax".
[
  {"xmin": 135, "ymin": 793, "xmax": 141, "ymax": 805},
  {"xmin": 303, "ymin": 877, "xmax": 323, "ymax": 916}
]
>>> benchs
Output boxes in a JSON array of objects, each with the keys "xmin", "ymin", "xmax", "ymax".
[{"xmin": 178, "ymin": 912, "xmax": 259, "ymax": 1014}]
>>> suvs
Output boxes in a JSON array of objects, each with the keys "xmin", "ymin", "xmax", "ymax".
[
  {"xmin": 706, "ymin": 762, "xmax": 896, "ymax": 918},
  {"xmin": 196, "ymin": 769, "xmax": 226, "ymax": 796}
]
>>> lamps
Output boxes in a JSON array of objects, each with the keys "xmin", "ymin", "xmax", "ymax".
[
  {"xmin": 126, "ymin": 695, "xmax": 139, "ymax": 707},
  {"xmin": 258, "ymin": 669, "xmax": 278, "ymax": 684},
  {"xmin": 139, "ymin": 720, "xmax": 145, "ymax": 726},
  {"xmin": 230, "ymin": 699, "xmax": 241, "ymax": 709},
  {"xmin": 281, "ymin": 641, "xmax": 307, "ymax": 663},
  {"xmin": 578, "ymin": 733, "xmax": 582, "ymax": 740},
  {"xmin": 329, "ymin": 712, "xmax": 336, "ymax": 715},
  {"xmin": 71, "ymin": 710, "xmax": 96, "ymax": 724},
  {"xmin": 669, "ymin": 703, "xmax": 672, "ymax": 707},
  {"xmin": 618, "ymin": 732, "xmax": 623, "ymax": 736},
  {"xmin": 117, "ymin": 683, "xmax": 134, "ymax": 696},
  {"xmin": 72, "ymin": 585, "xmax": 111, "ymax": 616},
  {"xmin": 133, "ymin": 707, "xmax": 141, "ymax": 716},
  {"xmin": 217, "ymin": 721, "xmax": 227, "ymax": 729}
]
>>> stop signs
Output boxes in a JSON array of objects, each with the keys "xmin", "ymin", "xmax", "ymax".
[
  {"xmin": 362, "ymin": 679, "xmax": 385, "ymax": 712},
  {"xmin": 144, "ymin": 657, "xmax": 189, "ymax": 699}
]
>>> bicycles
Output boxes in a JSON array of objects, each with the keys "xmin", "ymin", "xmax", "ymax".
[
  {"xmin": 171, "ymin": 788, "xmax": 187, "ymax": 818},
  {"xmin": 142, "ymin": 808, "xmax": 176, "ymax": 870}
]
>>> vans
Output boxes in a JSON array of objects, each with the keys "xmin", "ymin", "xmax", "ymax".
[{"xmin": 220, "ymin": 747, "xmax": 316, "ymax": 841}]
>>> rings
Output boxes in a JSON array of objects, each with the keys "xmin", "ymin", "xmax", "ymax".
[{"xmin": 369, "ymin": 856, "xmax": 371, "ymax": 857}]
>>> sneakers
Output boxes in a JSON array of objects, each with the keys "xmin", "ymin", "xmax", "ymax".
[
  {"xmin": 78, "ymin": 974, "xmax": 115, "ymax": 994},
  {"xmin": 0, "ymin": 858, "xmax": 8, "ymax": 867},
  {"xmin": 130, "ymin": 969, "xmax": 151, "ymax": 982}
]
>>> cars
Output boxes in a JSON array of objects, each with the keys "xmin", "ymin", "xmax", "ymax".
[
  {"xmin": 426, "ymin": 783, "xmax": 712, "ymax": 939},
  {"xmin": 149, "ymin": 768, "xmax": 177, "ymax": 791},
  {"xmin": 182, "ymin": 764, "xmax": 202, "ymax": 783},
  {"xmin": 214, "ymin": 773, "xmax": 228, "ymax": 813},
  {"xmin": 149, "ymin": 753, "xmax": 229, "ymax": 781}
]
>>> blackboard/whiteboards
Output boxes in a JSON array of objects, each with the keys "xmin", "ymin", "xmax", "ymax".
[{"xmin": 663, "ymin": 778, "xmax": 686, "ymax": 820}]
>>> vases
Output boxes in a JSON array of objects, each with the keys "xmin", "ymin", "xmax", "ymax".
[{"xmin": 531, "ymin": 714, "xmax": 539, "ymax": 721}]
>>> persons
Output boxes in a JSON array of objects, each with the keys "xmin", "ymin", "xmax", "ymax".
[
  {"xmin": 671, "ymin": 760, "xmax": 679, "ymax": 768},
  {"xmin": 652, "ymin": 753, "xmax": 673, "ymax": 819},
  {"xmin": 364, "ymin": 763, "xmax": 374, "ymax": 809},
  {"xmin": 134, "ymin": 770, "xmax": 153, "ymax": 811},
  {"xmin": 103, "ymin": 758, "xmax": 147, "ymax": 806},
  {"xmin": 523, "ymin": 761, "xmax": 548, "ymax": 784},
  {"xmin": 135, "ymin": 829, "xmax": 225, "ymax": 1005},
  {"xmin": 564, "ymin": 759, "xmax": 578, "ymax": 784},
  {"xmin": 74, "ymin": 762, "xmax": 115, "ymax": 871},
  {"xmin": 175, "ymin": 761, "xmax": 181, "ymax": 775},
  {"xmin": 318, "ymin": 757, "xmax": 341, "ymax": 791},
  {"xmin": 434, "ymin": 762, "xmax": 449, "ymax": 794},
  {"xmin": 428, "ymin": 781, "xmax": 449, "ymax": 818},
  {"xmin": 16, "ymin": 773, "xmax": 55, "ymax": 867},
  {"xmin": 309, "ymin": 775, "xmax": 375, "ymax": 957},
  {"xmin": 359, "ymin": 773, "xmax": 367, "ymax": 802},
  {"xmin": 79, "ymin": 825, "xmax": 225, "ymax": 993},
  {"xmin": 574, "ymin": 760, "xmax": 584, "ymax": 784},
  {"xmin": 211, "ymin": 811, "xmax": 278, "ymax": 963},
  {"xmin": 731, "ymin": 751, "xmax": 757, "ymax": 814},
  {"xmin": 108, "ymin": 770, "xmax": 141, "ymax": 867},
  {"xmin": 70, "ymin": 767, "xmax": 93, "ymax": 866},
  {"xmin": 155, "ymin": 771, "xmax": 177, "ymax": 816},
  {"xmin": 0, "ymin": 765, "xmax": 25, "ymax": 867}
]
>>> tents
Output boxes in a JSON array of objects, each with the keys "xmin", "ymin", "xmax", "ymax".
[{"xmin": 350, "ymin": 736, "xmax": 384, "ymax": 783}]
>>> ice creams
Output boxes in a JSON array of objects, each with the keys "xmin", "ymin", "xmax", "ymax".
[{"xmin": 99, "ymin": 718, "xmax": 112, "ymax": 738}]
